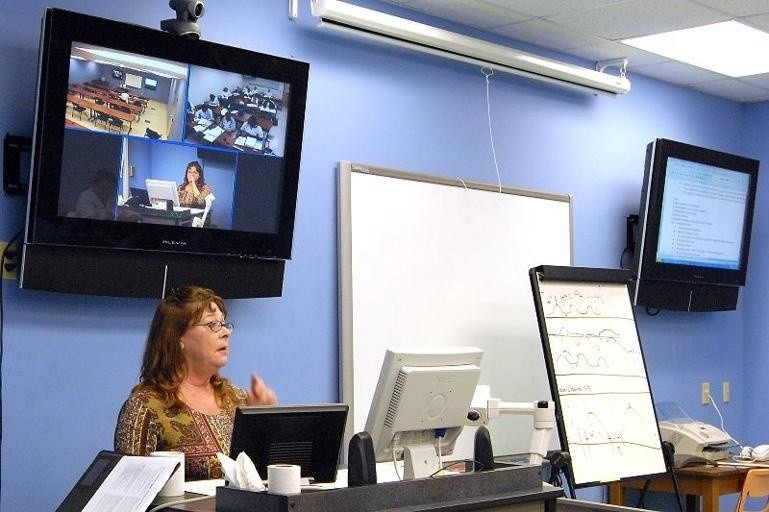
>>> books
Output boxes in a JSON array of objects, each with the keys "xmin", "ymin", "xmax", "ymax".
[{"xmin": 191, "ymin": 118, "xmax": 263, "ymax": 152}]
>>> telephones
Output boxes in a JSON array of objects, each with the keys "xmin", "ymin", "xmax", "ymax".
[{"xmin": 752, "ymin": 445, "xmax": 769, "ymax": 461}]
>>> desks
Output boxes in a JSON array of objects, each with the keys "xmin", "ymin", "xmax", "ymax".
[
  {"xmin": 187, "ymin": 92, "xmax": 277, "ymax": 150},
  {"xmin": 607, "ymin": 452, "xmax": 769, "ymax": 511},
  {"xmin": 120, "ymin": 203, "xmax": 206, "ymax": 227}
]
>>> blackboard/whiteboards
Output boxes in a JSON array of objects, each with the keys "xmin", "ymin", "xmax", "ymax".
[
  {"xmin": 529, "ymin": 266, "xmax": 668, "ymax": 490},
  {"xmin": 338, "ymin": 160, "xmax": 574, "ymax": 467}
]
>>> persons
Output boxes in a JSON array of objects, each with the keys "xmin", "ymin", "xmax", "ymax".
[
  {"xmin": 113, "ymin": 285, "xmax": 278, "ymax": 481},
  {"xmin": 119, "ymin": 88, "xmax": 130, "ymax": 105},
  {"xmin": 177, "ymin": 160, "xmax": 211, "ymax": 210},
  {"xmin": 185, "ymin": 83, "xmax": 278, "ymax": 141},
  {"xmin": 75, "ymin": 170, "xmax": 140, "ymax": 223}
]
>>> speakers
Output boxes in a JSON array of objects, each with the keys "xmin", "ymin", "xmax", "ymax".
[
  {"xmin": 474, "ymin": 426, "xmax": 495, "ymax": 472},
  {"xmin": 347, "ymin": 431, "xmax": 377, "ymax": 486}
]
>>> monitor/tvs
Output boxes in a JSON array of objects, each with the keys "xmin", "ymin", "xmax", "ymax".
[
  {"xmin": 221, "ymin": 402, "xmax": 349, "ymax": 483},
  {"xmin": 631, "ymin": 137, "xmax": 769, "ymax": 314},
  {"xmin": 16, "ymin": 5, "xmax": 310, "ymax": 299},
  {"xmin": 362, "ymin": 347, "xmax": 483, "ymax": 481}
]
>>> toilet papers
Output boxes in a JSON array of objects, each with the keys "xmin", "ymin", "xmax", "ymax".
[{"xmin": 267, "ymin": 461, "xmax": 302, "ymax": 495}]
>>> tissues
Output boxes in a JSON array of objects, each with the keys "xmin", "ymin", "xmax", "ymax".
[{"xmin": 216, "ymin": 452, "xmax": 283, "ymax": 511}]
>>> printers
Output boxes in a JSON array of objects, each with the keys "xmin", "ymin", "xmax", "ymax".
[{"xmin": 657, "ymin": 403, "xmax": 748, "ymax": 468}]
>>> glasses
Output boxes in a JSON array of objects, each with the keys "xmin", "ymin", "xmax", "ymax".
[{"xmin": 193, "ymin": 319, "xmax": 234, "ymax": 334}]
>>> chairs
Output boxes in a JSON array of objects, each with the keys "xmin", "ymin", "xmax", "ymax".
[{"xmin": 67, "ymin": 82, "xmax": 151, "ymax": 134}]
